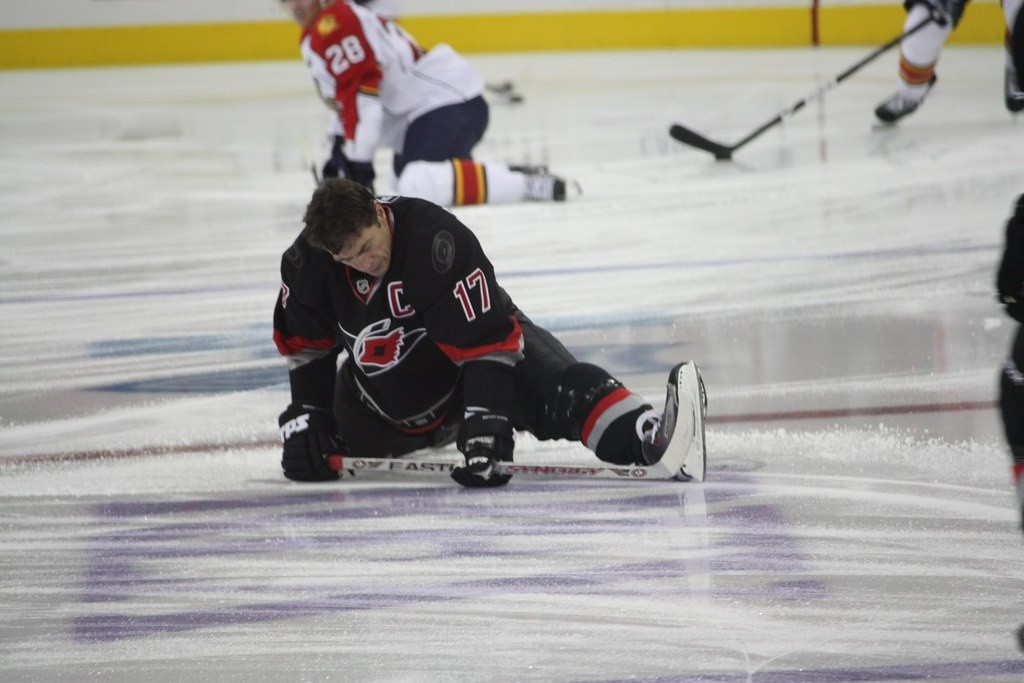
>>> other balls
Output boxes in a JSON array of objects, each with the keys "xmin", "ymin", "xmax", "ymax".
[{"xmin": 715, "ymin": 153, "xmax": 731, "ymax": 160}]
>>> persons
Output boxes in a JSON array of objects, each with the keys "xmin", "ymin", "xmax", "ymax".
[
  {"xmin": 873, "ymin": 0, "xmax": 1024, "ymax": 129},
  {"xmin": 286, "ymin": 1, "xmax": 584, "ymax": 207},
  {"xmin": 273, "ymin": 178, "xmax": 708, "ymax": 487},
  {"xmin": 990, "ymin": 194, "xmax": 1024, "ymax": 647}
]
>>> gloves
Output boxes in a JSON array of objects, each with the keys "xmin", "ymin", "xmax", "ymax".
[
  {"xmin": 278, "ymin": 400, "xmax": 344, "ymax": 482},
  {"xmin": 322, "ymin": 134, "xmax": 377, "ymax": 195},
  {"xmin": 450, "ymin": 404, "xmax": 515, "ymax": 487}
]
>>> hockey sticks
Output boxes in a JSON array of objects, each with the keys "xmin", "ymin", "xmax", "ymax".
[
  {"xmin": 324, "ymin": 361, "xmax": 698, "ymax": 481},
  {"xmin": 668, "ymin": 18, "xmax": 931, "ymax": 161}
]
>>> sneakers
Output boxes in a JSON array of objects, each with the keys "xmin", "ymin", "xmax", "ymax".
[
  {"xmin": 1004, "ymin": 64, "xmax": 1024, "ymax": 113},
  {"xmin": 512, "ymin": 162, "xmax": 582, "ymax": 202},
  {"xmin": 636, "ymin": 361, "xmax": 709, "ymax": 483},
  {"xmin": 871, "ymin": 73, "xmax": 936, "ymax": 131}
]
